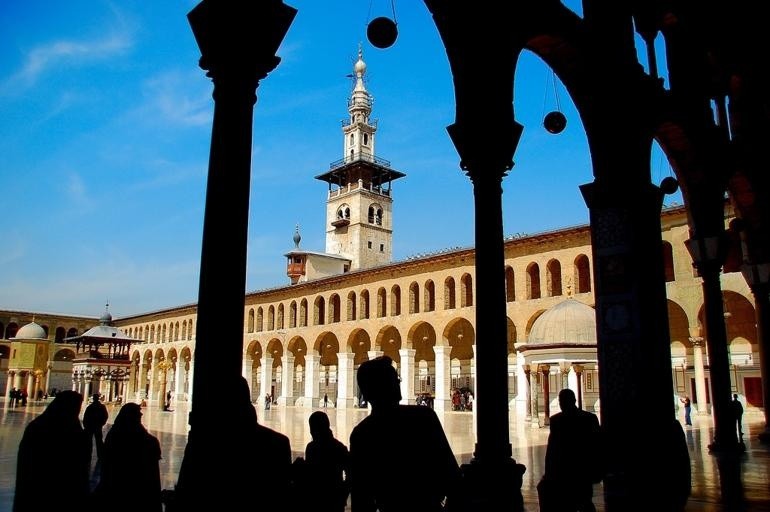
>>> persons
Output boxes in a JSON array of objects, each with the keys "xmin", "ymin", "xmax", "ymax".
[
  {"xmin": 733, "ymin": 394, "xmax": 744, "ymax": 436},
  {"xmin": 680, "ymin": 395, "xmax": 692, "ymax": 426}
]
